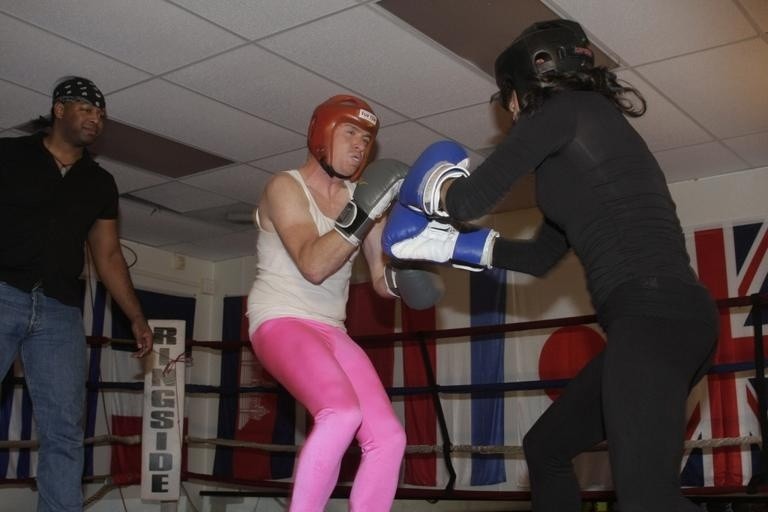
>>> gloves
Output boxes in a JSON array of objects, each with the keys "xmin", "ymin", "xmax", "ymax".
[
  {"xmin": 399, "ymin": 141, "xmax": 471, "ymax": 224},
  {"xmin": 333, "ymin": 158, "xmax": 411, "ymax": 247},
  {"xmin": 383, "ymin": 261, "xmax": 446, "ymax": 311},
  {"xmin": 381, "ymin": 201, "xmax": 499, "ymax": 272}
]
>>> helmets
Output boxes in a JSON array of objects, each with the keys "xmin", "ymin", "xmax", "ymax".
[
  {"xmin": 306, "ymin": 94, "xmax": 379, "ymax": 182},
  {"xmin": 494, "ymin": 18, "xmax": 594, "ymax": 110}
]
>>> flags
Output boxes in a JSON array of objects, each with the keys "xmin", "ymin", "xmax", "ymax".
[
  {"xmin": 3, "ymin": 285, "xmax": 196, "ymax": 486},
  {"xmin": 212, "ymin": 281, "xmax": 437, "ymax": 504},
  {"xmin": 467, "ymin": 223, "xmax": 768, "ymax": 495}
]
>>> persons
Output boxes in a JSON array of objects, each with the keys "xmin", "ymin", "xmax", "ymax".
[
  {"xmin": 241, "ymin": 91, "xmax": 444, "ymax": 512},
  {"xmin": 3, "ymin": 76, "xmax": 154, "ymax": 512},
  {"xmin": 381, "ymin": 18, "xmax": 720, "ymax": 511}
]
